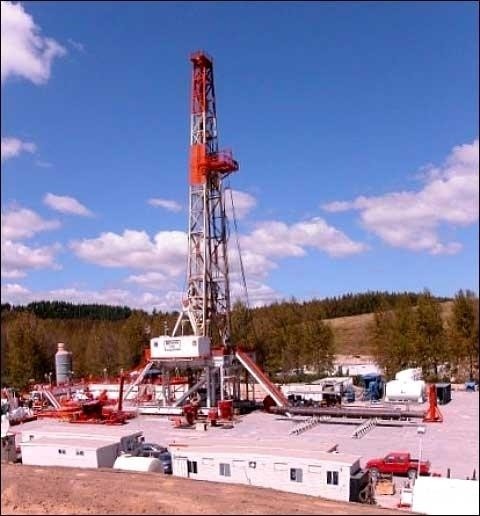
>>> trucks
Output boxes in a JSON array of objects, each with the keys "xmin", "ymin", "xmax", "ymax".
[{"xmin": 320, "ymin": 377, "xmax": 357, "ymax": 405}]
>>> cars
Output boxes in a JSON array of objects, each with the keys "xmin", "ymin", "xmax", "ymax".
[
  {"xmin": 133, "ymin": 440, "xmax": 172, "ymax": 473},
  {"xmin": 465, "ymin": 382, "xmax": 475, "ymax": 392}
]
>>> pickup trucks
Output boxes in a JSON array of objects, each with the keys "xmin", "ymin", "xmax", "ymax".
[{"xmin": 366, "ymin": 453, "xmax": 430, "ymax": 479}]
